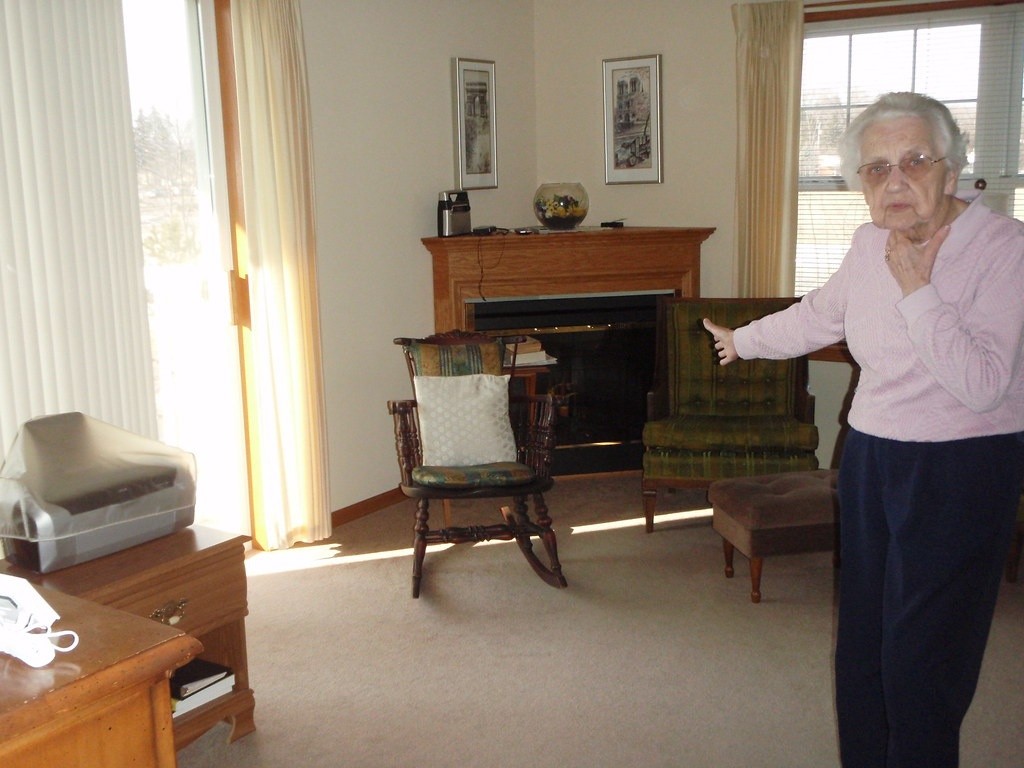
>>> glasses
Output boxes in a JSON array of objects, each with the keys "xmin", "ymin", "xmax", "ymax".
[{"xmin": 856, "ymin": 155, "xmax": 949, "ymax": 185}]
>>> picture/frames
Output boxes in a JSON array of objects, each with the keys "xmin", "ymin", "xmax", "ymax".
[
  {"xmin": 452, "ymin": 56, "xmax": 498, "ymax": 190},
  {"xmin": 602, "ymin": 53, "xmax": 662, "ymax": 185}
]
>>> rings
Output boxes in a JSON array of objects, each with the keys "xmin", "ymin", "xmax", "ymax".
[{"xmin": 884, "ymin": 248, "xmax": 892, "ymax": 261}]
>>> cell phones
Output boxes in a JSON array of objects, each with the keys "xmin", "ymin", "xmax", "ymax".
[{"xmin": 514, "ymin": 228, "xmax": 533, "ymax": 234}]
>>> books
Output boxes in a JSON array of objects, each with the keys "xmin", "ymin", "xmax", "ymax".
[
  {"xmin": 503, "ymin": 336, "xmax": 558, "ymax": 367},
  {"xmin": 171, "ymin": 658, "xmax": 236, "ymax": 719}
]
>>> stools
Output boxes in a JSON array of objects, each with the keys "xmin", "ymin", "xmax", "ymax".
[{"xmin": 708, "ymin": 469, "xmax": 837, "ymax": 604}]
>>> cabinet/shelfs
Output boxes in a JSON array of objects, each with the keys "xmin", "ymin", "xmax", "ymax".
[
  {"xmin": 1, "ymin": 575, "xmax": 204, "ymax": 768},
  {"xmin": 0, "ymin": 524, "xmax": 257, "ymax": 755}
]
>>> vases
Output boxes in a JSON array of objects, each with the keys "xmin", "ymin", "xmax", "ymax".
[{"xmin": 534, "ymin": 182, "xmax": 590, "ymax": 230}]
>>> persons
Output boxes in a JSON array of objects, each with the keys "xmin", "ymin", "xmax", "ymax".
[{"xmin": 703, "ymin": 91, "xmax": 1024, "ymax": 768}]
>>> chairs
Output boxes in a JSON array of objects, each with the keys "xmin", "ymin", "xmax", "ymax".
[
  {"xmin": 387, "ymin": 329, "xmax": 583, "ymax": 598},
  {"xmin": 643, "ymin": 294, "xmax": 818, "ymax": 533}
]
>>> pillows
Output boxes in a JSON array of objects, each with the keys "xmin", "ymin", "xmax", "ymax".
[{"xmin": 413, "ymin": 374, "xmax": 516, "ymax": 467}]
